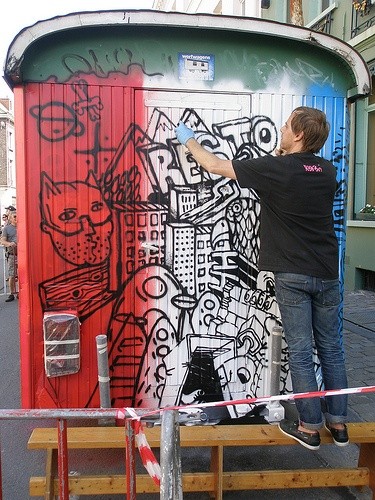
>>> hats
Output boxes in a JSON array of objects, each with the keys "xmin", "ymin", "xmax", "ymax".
[{"xmin": 5, "ymin": 206, "xmax": 17, "ymax": 211}]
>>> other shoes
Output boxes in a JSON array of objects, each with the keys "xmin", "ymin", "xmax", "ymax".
[{"xmin": 4, "ymin": 295, "xmax": 15, "ymax": 302}]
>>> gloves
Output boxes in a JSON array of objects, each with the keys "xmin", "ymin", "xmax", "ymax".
[{"xmin": 173, "ymin": 121, "xmax": 194, "ymax": 146}]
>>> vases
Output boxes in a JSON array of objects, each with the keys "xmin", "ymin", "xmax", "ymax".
[{"xmin": 360, "ymin": 213, "xmax": 375, "ymax": 221}]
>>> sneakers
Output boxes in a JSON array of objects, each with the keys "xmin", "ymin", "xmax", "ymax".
[
  {"xmin": 278, "ymin": 418, "xmax": 321, "ymax": 450},
  {"xmin": 323, "ymin": 420, "xmax": 349, "ymax": 446}
]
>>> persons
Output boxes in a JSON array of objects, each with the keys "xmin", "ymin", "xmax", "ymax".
[
  {"xmin": 175, "ymin": 106, "xmax": 350, "ymax": 449},
  {"xmin": 0, "ymin": 206, "xmax": 20, "ymax": 303}
]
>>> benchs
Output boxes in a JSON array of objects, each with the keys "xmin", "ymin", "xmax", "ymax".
[{"xmin": 28, "ymin": 422, "xmax": 375, "ymax": 500}]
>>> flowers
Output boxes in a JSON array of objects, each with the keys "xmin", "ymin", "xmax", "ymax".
[{"xmin": 359, "ymin": 204, "xmax": 375, "ymax": 214}]
